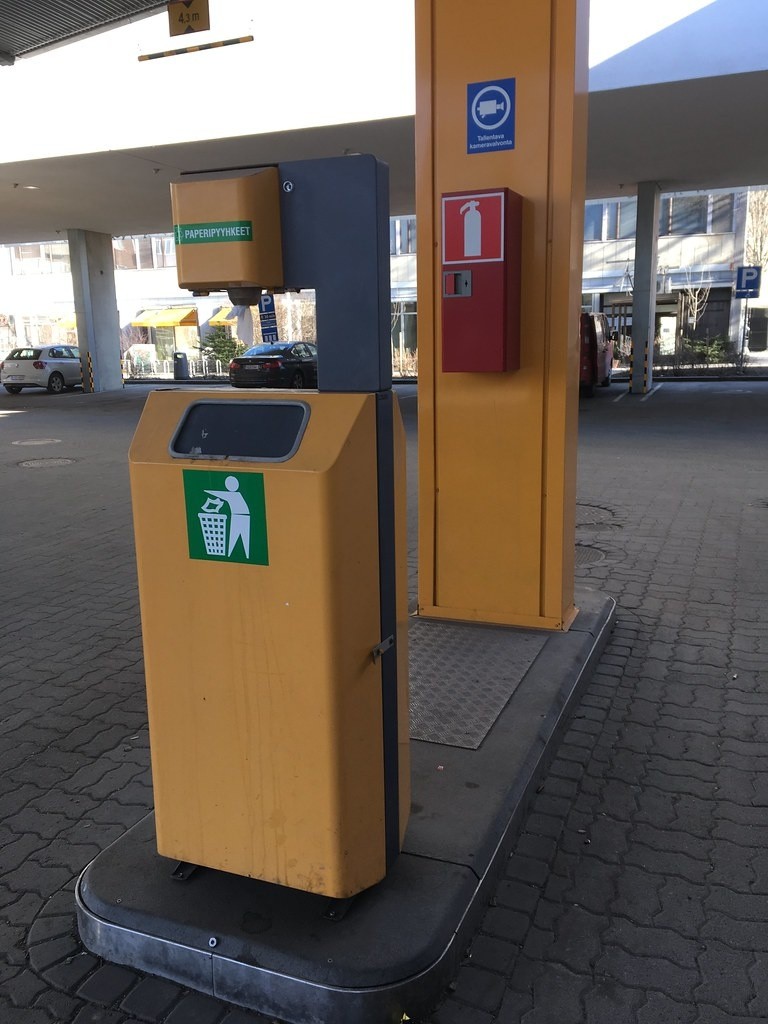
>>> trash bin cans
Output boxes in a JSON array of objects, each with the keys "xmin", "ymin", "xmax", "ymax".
[
  {"xmin": 174, "ymin": 352, "xmax": 188, "ymax": 380},
  {"xmin": 127, "ymin": 150, "xmax": 412, "ymax": 897}
]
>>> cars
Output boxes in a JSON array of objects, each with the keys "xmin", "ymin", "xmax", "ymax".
[
  {"xmin": 0, "ymin": 346, "xmax": 82, "ymax": 394},
  {"xmin": 230, "ymin": 341, "xmax": 317, "ymax": 388}
]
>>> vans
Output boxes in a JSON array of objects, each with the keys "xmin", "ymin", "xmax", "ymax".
[{"xmin": 581, "ymin": 313, "xmax": 619, "ymax": 388}]
